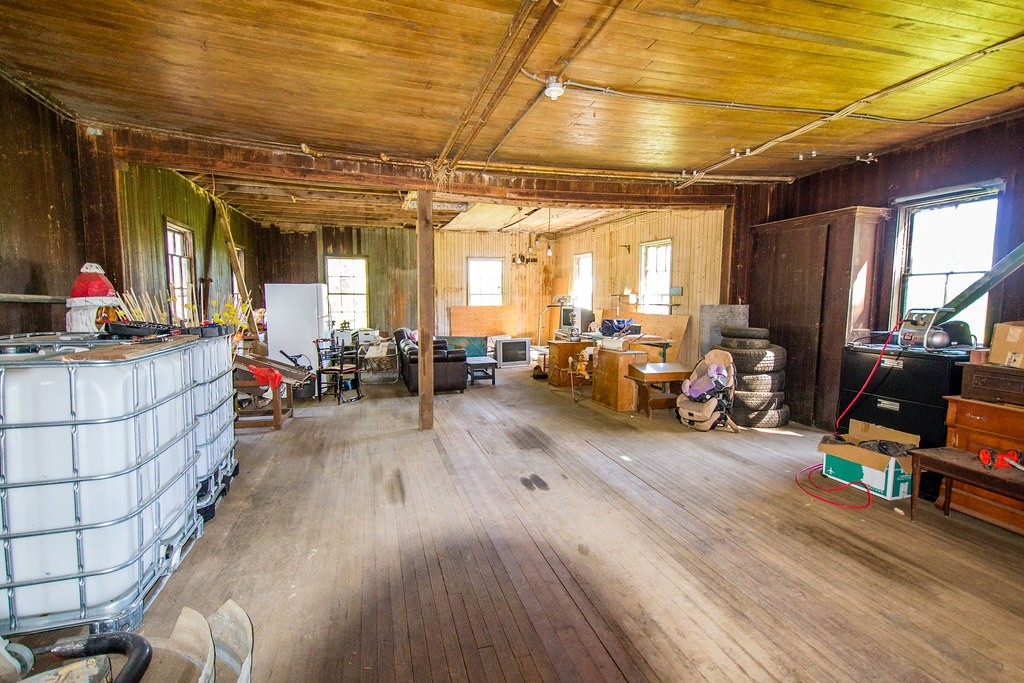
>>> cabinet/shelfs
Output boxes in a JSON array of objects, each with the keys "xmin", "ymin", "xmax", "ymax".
[
  {"xmin": 548, "ymin": 340, "xmax": 594, "ymax": 386},
  {"xmin": 591, "ymin": 347, "xmax": 648, "ymax": 413},
  {"xmin": 934, "ymin": 395, "xmax": 1024, "ymax": 536},
  {"xmin": 835, "ymin": 345, "xmax": 969, "ymax": 500},
  {"xmin": 749, "ymin": 207, "xmax": 894, "ymax": 431}
]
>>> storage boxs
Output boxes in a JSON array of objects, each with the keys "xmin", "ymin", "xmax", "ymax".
[
  {"xmin": 987, "ymin": 321, "xmax": 1024, "ymax": 369},
  {"xmin": 381, "ymin": 332, "xmax": 388, "ymax": 337},
  {"xmin": 956, "ymin": 362, "xmax": 1024, "ymax": 406},
  {"xmin": 334, "ymin": 329, "xmax": 358, "ymax": 345},
  {"xmin": 818, "ymin": 419, "xmax": 928, "ymax": 501},
  {"xmin": 359, "ymin": 329, "xmax": 379, "ymax": 344}
]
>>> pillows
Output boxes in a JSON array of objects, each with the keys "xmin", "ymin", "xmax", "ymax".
[{"xmin": 411, "ymin": 330, "xmax": 418, "ymax": 341}]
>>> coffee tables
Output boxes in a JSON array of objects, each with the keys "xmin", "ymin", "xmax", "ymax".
[{"xmin": 466, "ymin": 356, "xmax": 497, "ymax": 386}]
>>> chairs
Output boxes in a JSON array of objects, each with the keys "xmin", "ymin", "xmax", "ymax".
[{"xmin": 313, "ymin": 336, "xmax": 363, "ymax": 403}]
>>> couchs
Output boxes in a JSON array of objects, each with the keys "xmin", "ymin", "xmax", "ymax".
[{"xmin": 394, "ymin": 327, "xmax": 468, "ymax": 397}]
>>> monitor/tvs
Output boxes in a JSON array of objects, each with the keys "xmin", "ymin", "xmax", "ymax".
[
  {"xmin": 559, "ymin": 305, "xmax": 595, "ymax": 335},
  {"xmin": 493, "ymin": 338, "xmax": 531, "ymax": 368}
]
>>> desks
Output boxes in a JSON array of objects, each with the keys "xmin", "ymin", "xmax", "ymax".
[
  {"xmin": 624, "ymin": 375, "xmax": 685, "ymax": 420},
  {"xmin": 232, "ymin": 380, "xmax": 294, "ymax": 430},
  {"xmin": 906, "ymin": 446, "xmax": 1024, "ymax": 523},
  {"xmin": 582, "ymin": 332, "xmax": 678, "ymax": 394},
  {"xmin": 243, "ymin": 333, "xmax": 265, "ymax": 354},
  {"xmin": 338, "ymin": 337, "xmax": 392, "ymax": 372}
]
